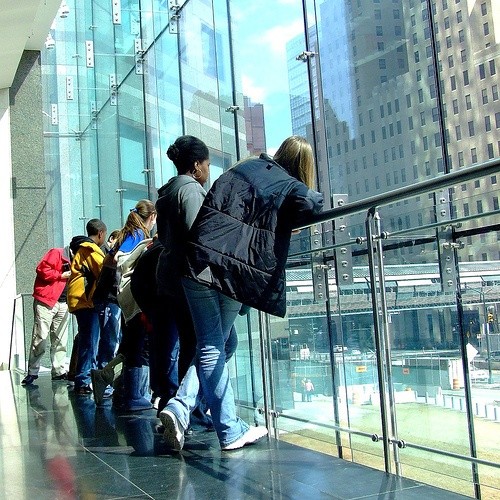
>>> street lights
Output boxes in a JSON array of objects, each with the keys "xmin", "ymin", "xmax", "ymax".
[{"xmin": 460, "ymin": 282, "xmax": 497, "ymax": 385}]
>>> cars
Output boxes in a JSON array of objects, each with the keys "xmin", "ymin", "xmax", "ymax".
[{"xmin": 334, "ymin": 345, "xmax": 347, "ymax": 353}]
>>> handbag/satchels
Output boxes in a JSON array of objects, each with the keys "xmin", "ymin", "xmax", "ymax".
[{"xmin": 129, "ymin": 242, "xmax": 194, "ymax": 333}]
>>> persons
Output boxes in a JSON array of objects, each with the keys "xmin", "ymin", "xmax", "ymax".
[
  {"xmin": 300, "ymin": 378, "xmax": 314, "ymax": 402},
  {"xmin": 67, "ymin": 136, "xmax": 237, "ymax": 434},
  {"xmin": 21, "ymin": 247, "xmax": 73, "ymax": 384},
  {"xmin": 160, "ymin": 136, "xmax": 325, "ymax": 451}
]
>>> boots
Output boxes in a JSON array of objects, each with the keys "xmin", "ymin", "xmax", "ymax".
[
  {"xmin": 123, "ymin": 367, "xmax": 153, "ymax": 411},
  {"xmin": 91, "ymin": 353, "xmax": 128, "ymax": 407}
]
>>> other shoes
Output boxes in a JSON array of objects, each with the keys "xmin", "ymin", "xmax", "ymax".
[
  {"xmin": 160, "ymin": 410, "xmax": 185, "ymax": 451},
  {"xmin": 21, "ymin": 375, "xmax": 38, "ymax": 384},
  {"xmin": 102, "ymin": 387, "xmax": 115, "ymax": 397},
  {"xmin": 222, "ymin": 426, "xmax": 268, "ymax": 449},
  {"xmin": 52, "ymin": 373, "xmax": 68, "ymax": 380},
  {"xmin": 67, "ymin": 381, "xmax": 75, "ymax": 385},
  {"xmin": 73, "ymin": 386, "xmax": 92, "ymax": 394}
]
